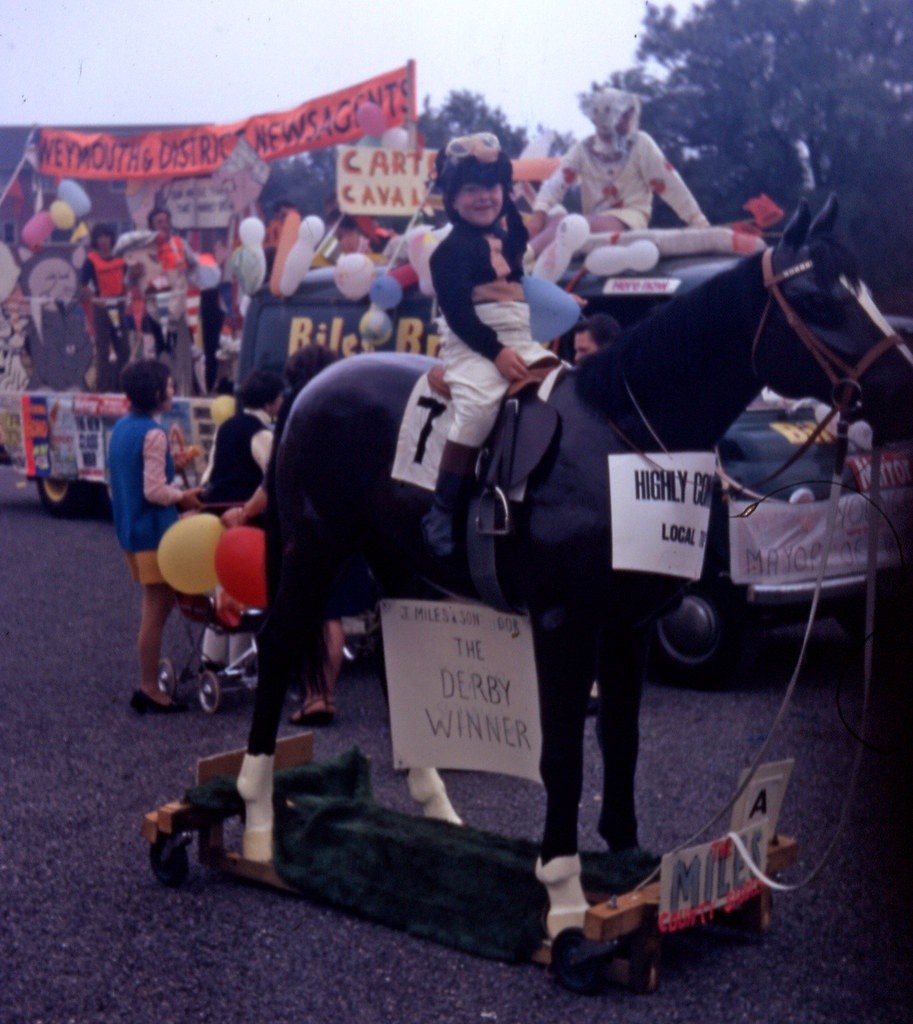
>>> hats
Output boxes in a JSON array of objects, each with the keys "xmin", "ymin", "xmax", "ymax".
[{"xmin": 435, "ymin": 145, "xmax": 513, "ymax": 225}]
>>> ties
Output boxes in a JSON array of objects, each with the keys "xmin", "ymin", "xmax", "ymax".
[{"xmin": 483, "ymin": 232, "xmax": 511, "ymax": 285}]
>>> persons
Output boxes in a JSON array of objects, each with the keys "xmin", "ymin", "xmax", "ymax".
[
  {"xmin": 575, "ymin": 316, "xmax": 623, "ymax": 717},
  {"xmin": 75, "ymin": 196, "xmax": 200, "ymax": 396},
  {"xmin": 419, "ymin": 134, "xmax": 570, "ymax": 557},
  {"xmin": 524, "ymin": 87, "xmax": 709, "ymax": 279},
  {"xmin": 108, "ymin": 361, "xmax": 206, "ymax": 713},
  {"xmin": 265, "ymin": 194, "xmax": 410, "ymax": 271},
  {"xmin": 197, "ymin": 345, "xmax": 344, "ymax": 721}
]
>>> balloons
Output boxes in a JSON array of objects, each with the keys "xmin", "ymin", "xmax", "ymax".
[
  {"xmin": 21, "ymin": 179, "xmax": 93, "ymax": 249},
  {"xmin": 354, "ymin": 103, "xmax": 409, "ymax": 150},
  {"xmin": 158, "ymin": 513, "xmax": 266, "ymax": 611},
  {"xmin": 239, "ymin": 213, "xmax": 660, "ymax": 352}
]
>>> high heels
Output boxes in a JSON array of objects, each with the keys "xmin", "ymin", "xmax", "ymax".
[
  {"xmin": 130, "ymin": 690, "xmax": 191, "ymax": 716},
  {"xmin": 288, "ymin": 697, "xmax": 337, "ymax": 728}
]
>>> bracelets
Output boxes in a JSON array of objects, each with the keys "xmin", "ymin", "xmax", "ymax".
[{"xmin": 239, "ymin": 506, "xmax": 248, "ymax": 520}]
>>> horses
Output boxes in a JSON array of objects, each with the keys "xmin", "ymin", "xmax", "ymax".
[{"xmin": 236, "ymin": 191, "xmax": 912, "ymax": 941}]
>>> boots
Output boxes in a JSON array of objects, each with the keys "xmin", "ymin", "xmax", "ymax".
[{"xmin": 423, "ymin": 440, "xmax": 479, "ymax": 567}]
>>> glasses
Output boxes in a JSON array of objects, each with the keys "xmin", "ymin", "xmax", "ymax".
[{"xmin": 442, "ymin": 132, "xmax": 502, "ymax": 166}]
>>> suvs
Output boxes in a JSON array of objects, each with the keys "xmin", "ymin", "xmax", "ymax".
[{"xmin": 231, "ymin": 249, "xmax": 913, "ymax": 693}]
